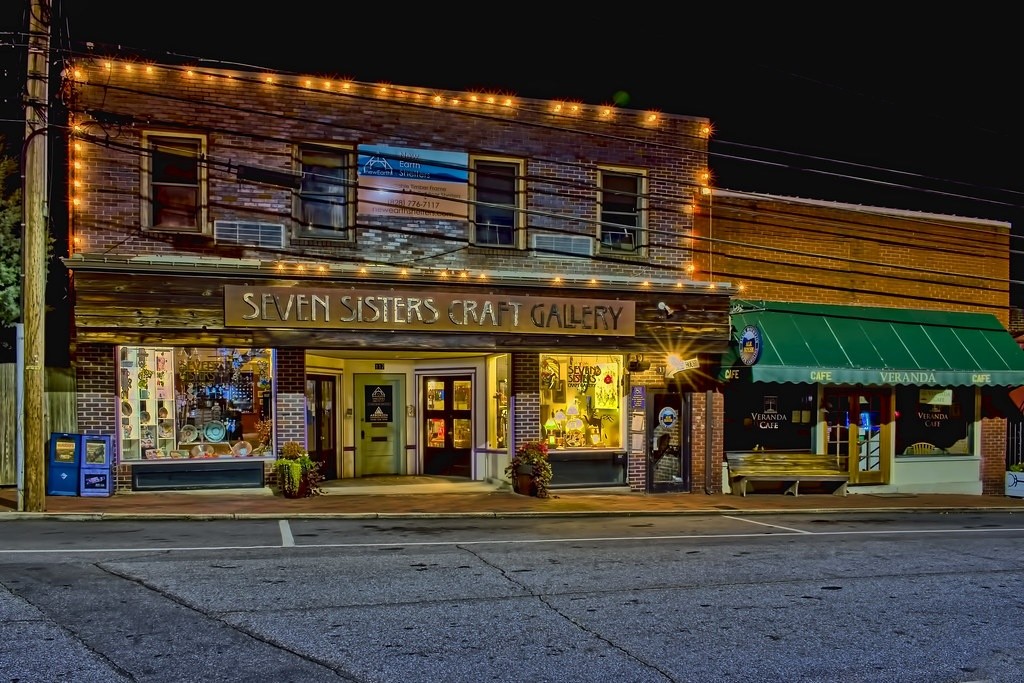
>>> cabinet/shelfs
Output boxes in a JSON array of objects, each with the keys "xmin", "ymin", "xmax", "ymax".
[{"xmin": 120, "ymin": 346, "xmax": 176, "ymax": 460}]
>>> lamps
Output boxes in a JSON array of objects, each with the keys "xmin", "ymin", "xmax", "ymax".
[
  {"xmin": 636, "ymin": 352, "xmax": 650, "ymax": 370},
  {"xmin": 658, "ymin": 302, "xmax": 675, "ymax": 315}
]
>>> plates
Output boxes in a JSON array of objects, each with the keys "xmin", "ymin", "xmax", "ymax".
[
  {"xmin": 122, "ymin": 402, "xmax": 132, "ymax": 416},
  {"xmin": 182, "ymin": 425, "xmax": 197, "ymax": 442},
  {"xmin": 233, "ymin": 441, "xmax": 252, "ymax": 456},
  {"xmin": 159, "ymin": 407, "xmax": 168, "ymax": 417},
  {"xmin": 205, "ymin": 421, "xmax": 225, "ymax": 442},
  {"xmin": 141, "ymin": 410, "xmax": 150, "ymax": 422},
  {"xmin": 158, "ymin": 422, "xmax": 173, "ymax": 437}
]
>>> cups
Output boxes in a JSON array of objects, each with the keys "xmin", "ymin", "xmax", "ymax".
[
  {"xmin": 158, "ymin": 390, "xmax": 167, "ymax": 398},
  {"xmin": 141, "ymin": 390, "xmax": 150, "ymax": 398},
  {"xmin": 121, "ymin": 391, "xmax": 128, "ymax": 399}
]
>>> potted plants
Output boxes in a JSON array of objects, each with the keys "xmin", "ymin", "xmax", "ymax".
[
  {"xmin": 1004, "ymin": 462, "xmax": 1024, "ymax": 497},
  {"xmin": 271, "ymin": 441, "xmax": 329, "ymax": 499}
]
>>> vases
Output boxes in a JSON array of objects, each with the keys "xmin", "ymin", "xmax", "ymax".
[{"xmin": 512, "ymin": 467, "xmax": 537, "ymax": 496}]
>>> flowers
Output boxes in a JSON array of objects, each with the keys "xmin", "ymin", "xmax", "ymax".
[{"xmin": 504, "ymin": 440, "xmax": 559, "ymax": 499}]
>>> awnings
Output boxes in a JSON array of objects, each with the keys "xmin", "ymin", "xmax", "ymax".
[{"xmin": 726, "ymin": 297, "xmax": 1024, "ymax": 389}]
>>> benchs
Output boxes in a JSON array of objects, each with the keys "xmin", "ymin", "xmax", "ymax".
[{"xmin": 726, "ymin": 452, "xmax": 851, "ymax": 497}]
